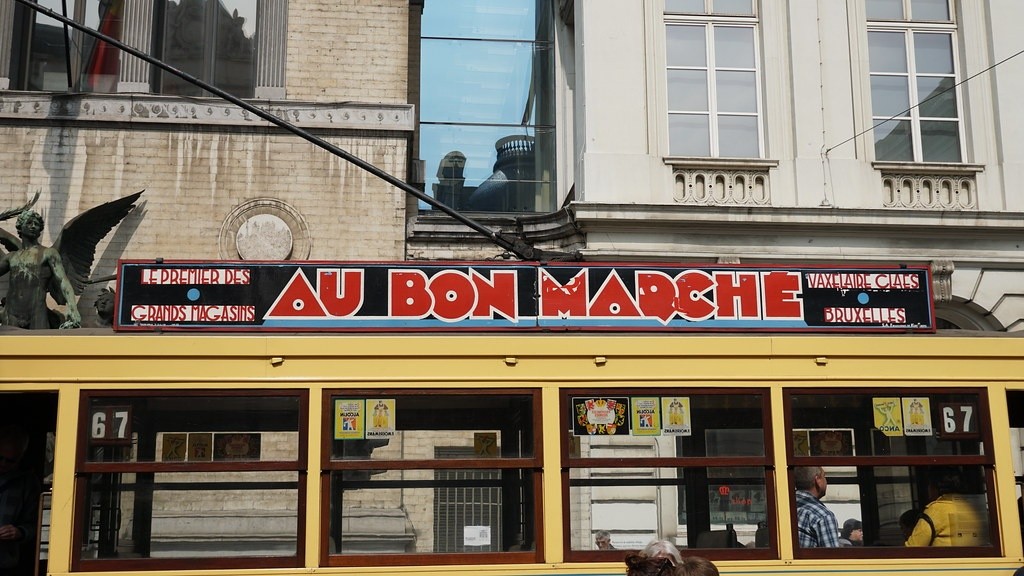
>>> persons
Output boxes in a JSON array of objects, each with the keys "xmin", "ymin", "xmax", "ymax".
[
  {"xmin": 595, "ymin": 530, "xmax": 615, "ymax": 550},
  {"xmin": 838, "ymin": 519, "xmax": 863, "ymax": 547},
  {"xmin": 795, "ymin": 466, "xmax": 840, "ymax": 548},
  {"xmin": 0, "ymin": 211, "xmax": 81, "ymax": 329},
  {"xmin": 0, "ymin": 423, "xmax": 41, "ymax": 576},
  {"xmin": 625, "ymin": 540, "xmax": 719, "ymax": 576},
  {"xmin": 93, "ymin": 286, "xmax": 116, "ymax": 328},
  {"xmin": 898, "ymin": 509, "xmax": 921, "ymax": 539},
  {"xmin": 904, "ymin": 465, "xmax": 982, "ymax": 547}
]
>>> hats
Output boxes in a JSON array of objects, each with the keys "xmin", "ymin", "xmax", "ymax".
[{"xmin": 841, "ymin": 519, "xmax": 862, "ymax": 539}]
